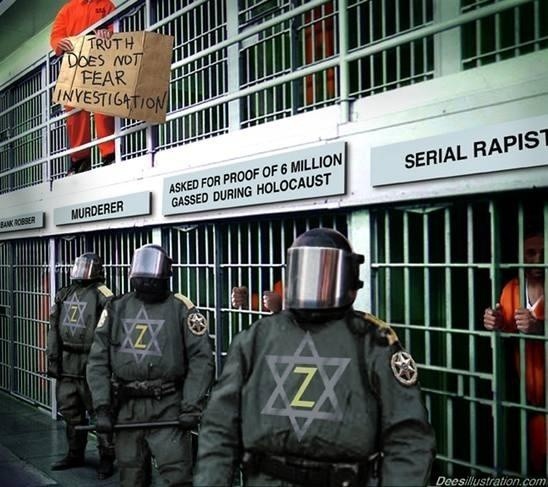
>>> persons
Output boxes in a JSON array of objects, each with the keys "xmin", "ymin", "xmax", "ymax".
[
  {"xmin": 86, "ymin": 243, "xmax": 215, "ymax": 486},
  {"xmin": 50, "ymin": 1, "xmax": 124, "ymax": 178},
  {"xmin": 189, "ymin": 227, "xmax": 441, "ymax": 486},
  {"xmin": 228, "ymin": 268, "xmax": 285, "ymax": 316},
  {"xmin": 483, "ymin": 230, "xmax": 546, "ymax": 483},
  {"xmin": 46, "ymin": 251, "xmax": 118, "ymax": 481},
  {"xmin": 296, "ymin": 0, "xmax": 336, "ymax": 104}
]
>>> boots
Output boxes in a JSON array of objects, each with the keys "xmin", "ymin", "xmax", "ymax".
[
  {"xmin": 97, "ymin": 458, "xmax": 112, "ymax": 479},
  {"xmin": 50, "ymin": 449, "xmax": 84, "ymax": 470}
]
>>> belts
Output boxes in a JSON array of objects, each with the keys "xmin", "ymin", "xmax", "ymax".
[
  {"xmin": 110, "ymin": 379, "xmax": 175, "ymax": 399},
  {"xmin": 253, "ymin": 453, "xmax": 384, "ymax": 486}
]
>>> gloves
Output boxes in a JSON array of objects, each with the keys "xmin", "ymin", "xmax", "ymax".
[
  {"xmin": 95, "ymin": 406, "xmax": 111, "ymax": 433},
  {"xmin": 47, "ymin": 359, "xmax": 61, "ymax": 380},
  {"xmin": 178, "ymin": 412, "xmax": 195, "ymax": 430}
]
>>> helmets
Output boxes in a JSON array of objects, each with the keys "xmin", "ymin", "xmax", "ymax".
[
  {"xmin": 284, "ymin": 228, "xmax": 363, "ymax": 319},
  {"xmin": 69, "ymin": 252, "xmax": 102, "ymax": 284},
  {"xmin": 129, "ymin": 244, "xmax": 173, "ymax": 298}
]
